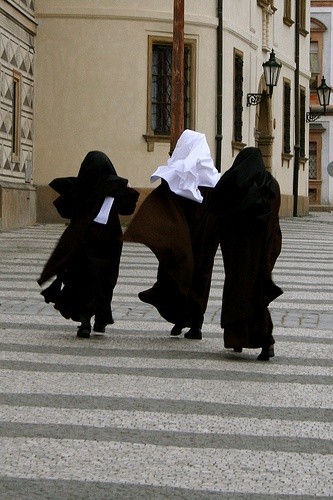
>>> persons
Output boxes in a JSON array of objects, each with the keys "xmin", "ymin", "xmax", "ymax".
[
  {"xmin": 122, "ymin": 130, "xmax": 220, "ymax": 339},
  {"xmin": 37, "ymin": 151, "xmax": 139, "ymax": 338},
  {"xmin": 206, "ymin": 146, "xmax": 284, "ymax": 361}
]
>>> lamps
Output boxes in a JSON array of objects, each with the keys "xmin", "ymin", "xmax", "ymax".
[
  {"xmin": 246, "ymin": 47, "xmax": 283, "ymax": 108},
  {"xmin": 306, "ymin": 74, "xmax": 332, "ymax": 123}
]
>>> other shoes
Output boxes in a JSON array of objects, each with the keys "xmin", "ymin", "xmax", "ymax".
[
  {"xmin": 257, "ymin": 348, "xmax": 274, "ymax": 360},
  {"xmin": 77, "ymin": 326, "xmax": 91, "ymax": 337},
  {"xmin": 184, "ymin": 329, "xmax": 202, "ymax": 339},
  {"xmin": 234, "ymin": 348, "xmax": 242, "ymax": 353},
  {"xmin": 171, "ymin": 322, "xmax": 184, "ymax": 335},
  {"xmin": 93, "ymin": 318, "xmax": 106, "ymax": 333}
]
